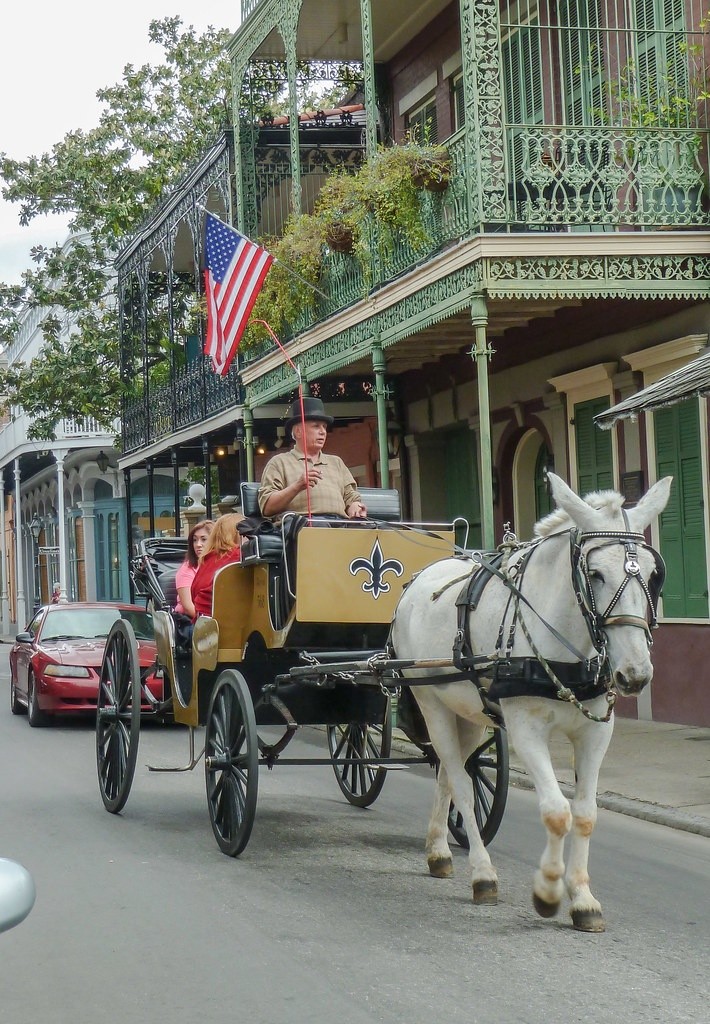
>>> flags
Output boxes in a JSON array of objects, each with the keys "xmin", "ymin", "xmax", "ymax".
[{"xmin": 203, "ymin": 212, "xmax": 274, "ymax": 376}]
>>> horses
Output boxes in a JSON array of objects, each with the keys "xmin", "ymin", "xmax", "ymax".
[{"xmin": 392, "ymin": 472, "xmax": 675, "ymax": 932}]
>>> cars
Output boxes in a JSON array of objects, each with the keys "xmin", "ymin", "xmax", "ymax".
[{"xmin": 9, "ymin": 602, "xmax": 164, "ymax": 727}]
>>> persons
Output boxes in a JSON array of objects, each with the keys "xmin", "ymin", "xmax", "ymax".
[
  {"xmin": 174, "ymin": 512, "xmax": 250, "ymax": 625},
  {"xmin": 258, "ymin": 398, "xmax": 389, "ymax": 547},
  {"xmin": 50, "ymin": 582, "xmax": 60, "ymax": 604}
]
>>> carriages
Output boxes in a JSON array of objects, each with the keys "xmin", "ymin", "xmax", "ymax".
[{"xmin": 96, "ymin": 319, "xmax": 673, "ymax": 932}]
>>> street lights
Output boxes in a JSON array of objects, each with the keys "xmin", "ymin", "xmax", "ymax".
[{"xmin": 30, "ymin": 513, "xmax": 43, "ymax": 616}]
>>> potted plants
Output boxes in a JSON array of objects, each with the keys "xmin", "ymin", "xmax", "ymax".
[{"xmin": 217, "ymin": 116, "xmax": 460, "ymax": 353}]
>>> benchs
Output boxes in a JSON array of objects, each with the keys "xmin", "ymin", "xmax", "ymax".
[
  {"xmin": 238, "ymin": 481, "xmax": 402, "ymax": 563},
  {"xmin": 159, "ymin": 569, "xmax": 181, "ymax": 618}
]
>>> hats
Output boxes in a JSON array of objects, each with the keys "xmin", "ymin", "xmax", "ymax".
[{"xmin": 284, "ymin": 398, "xmax": 334, "ymax": 442}]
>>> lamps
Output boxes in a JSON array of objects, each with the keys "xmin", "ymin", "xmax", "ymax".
[
  {"xmin": 274, "ymin": 427, "xmax": 285, "ymax": 448},
  {"xmin": 96, "ymin": 451, "xmax": 109, "ymax": 475},
  {"xmin": 228, "ymin": 441, "xmax": 240, "ymax": 454},
  {"xmin": 30, "ymin": 512, "xmax": 42, "ymax": 537}
]
